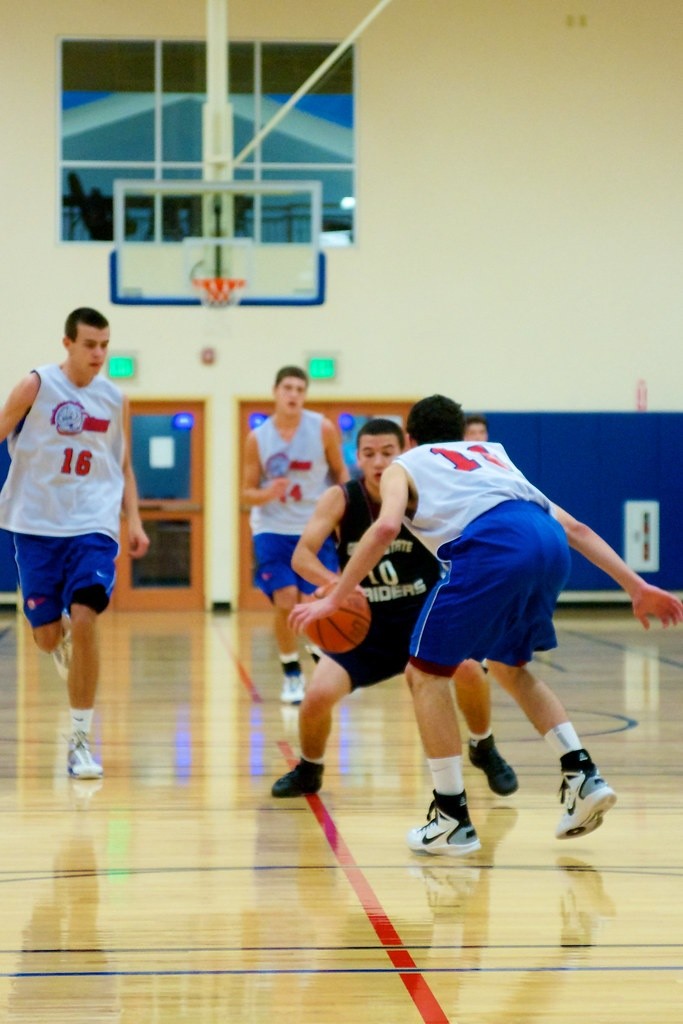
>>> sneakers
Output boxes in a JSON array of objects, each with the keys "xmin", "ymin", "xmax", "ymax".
[
  {"xmin": 468, "ymin": 735, "xmax": 519, "ymax": 796},
  {"xmin": 52, "ymin": 631, "xmax": 73, "ymax": 679},
  {"xmin": 555, "ymin": 765, "xmax": 617, "ymax": 839},
  {"xmin": 271, "ymin": 757, "xmax": 323, "ymax": 797},
  {"xmin": 407, "ymin": 800, "xmax": 481, "ymax": 856},
  {"xmin": 67, "ymin": 730, "xmax": 103, "ymax": 780}
]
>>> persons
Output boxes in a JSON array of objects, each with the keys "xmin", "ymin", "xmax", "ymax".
[
  {"xmin": 0, "ymin": 308, "xmax": 150, "ymax": 778},
  {"xmin": 464, "ymin": 416, "xmax": 488, "ymax": 442},
  {"xmin": 272, "ymin": 420, "xmax": 520, "ymax": 798},
  {"xmin": 241, "ymin": 366, "xmax": 352, "ymax": 703},
  {"xmin": 2, "ymin": 795, "xmax": 622, "ymax": 1024},
  {"xmin": 288, "ymin": 396, "xmax": 683, "ymax": 856}
]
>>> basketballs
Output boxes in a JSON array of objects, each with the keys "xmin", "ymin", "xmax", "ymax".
[{"xmin": 303, "ymin": 583, "xmax": 371, "ymax": 654}]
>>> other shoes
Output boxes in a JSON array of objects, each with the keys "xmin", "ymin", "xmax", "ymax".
[{"xmin": 282, "ymin": 674, "xmax": 305, "ymax": 704}]
